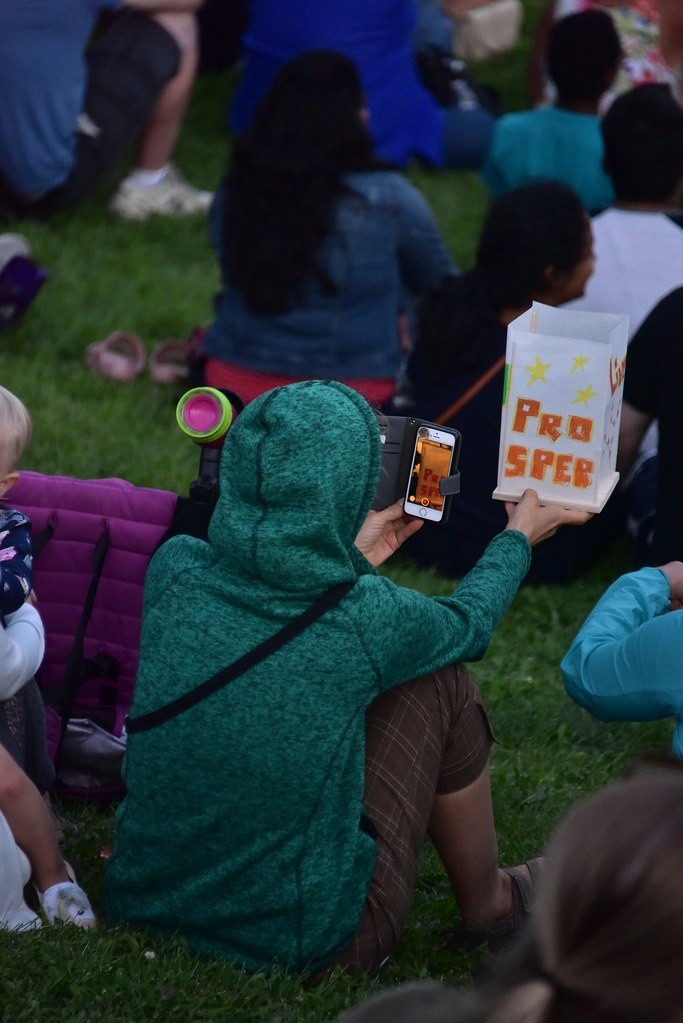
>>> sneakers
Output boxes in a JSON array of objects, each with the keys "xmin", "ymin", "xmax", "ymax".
[{"xmin": 108, "ymin": 169, "xmax": 216, "ymax": 221}]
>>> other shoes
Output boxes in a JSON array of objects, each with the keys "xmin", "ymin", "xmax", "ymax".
[
  {"xmin": 29, "ymin": 858, "xmax": 98, "ymax": 934},
  {"xmin": 466, "ymin": 854, "xmax": 550, "ymax": 942}
]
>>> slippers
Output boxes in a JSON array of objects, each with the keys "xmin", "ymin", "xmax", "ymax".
[{"xmin": 82, "ymin": 329, "xmax": 143, "ymax": 383}]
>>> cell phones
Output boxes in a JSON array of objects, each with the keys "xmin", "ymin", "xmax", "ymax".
[{"xmin": 401, "ymin": 424, "xmax": 459, "ymax": 522}]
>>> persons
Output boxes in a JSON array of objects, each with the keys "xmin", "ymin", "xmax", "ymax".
[
  {"xmin": 96, "ymin": 378, "xmax": 591, "ymax": 985},
  {"xmin": 0, "ymin": 1, "xmax": 683, "ymax": 1023}
]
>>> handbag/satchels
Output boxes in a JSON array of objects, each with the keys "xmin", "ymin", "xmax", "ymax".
[
  {"xmin": 450, "ymin": 1, "xmax": 521, "ymax": 63},
  {"xmin": 149, "ymin": 337, "xmax": 193, "ymax": 383},
  {"xmin": 55, "ymin": 720, "xmax": 131, "ymax": 803}
]
